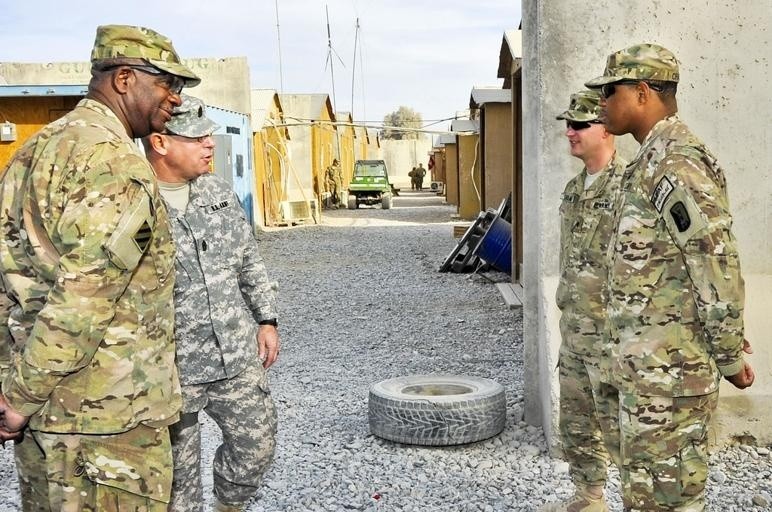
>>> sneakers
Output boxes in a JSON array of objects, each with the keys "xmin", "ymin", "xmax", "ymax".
[{"xmin": 540, "ymin": 487, "xmax": 608, "ymax": 512}]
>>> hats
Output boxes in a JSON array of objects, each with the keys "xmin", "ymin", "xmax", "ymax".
[
  {"xmin": 584, "ymin": 42, "xmax": 679, "ymax": 89},
  {"xmin": 91, "ymin": 23, "xmax": 201, "ymax": 89},
  {"xmin": 555, "ymin": 90, "xmax": 606, "ymax": 123},
  {"xmin": 161, "ymin": 92, "xmax": 221, "ymax": 138}
]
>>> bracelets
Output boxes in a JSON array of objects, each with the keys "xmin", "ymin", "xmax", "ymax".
[{"xmin": 258, "ymin": 315, "xmax": 277, "ymax": 326}]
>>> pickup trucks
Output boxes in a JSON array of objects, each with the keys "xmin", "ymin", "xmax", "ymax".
[{"xmin": 347, "ymin": 161, "xmax": 392, "ymax": 209}]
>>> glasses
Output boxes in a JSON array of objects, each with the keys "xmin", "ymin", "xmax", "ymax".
[
  {"xmin": 601, "ymin": 80, "xmax": 664, "ymax": 99},
  {"xmin": 566, "ymin": 118, "xmax": 602, "ymax": 131},
  {"xmin": 132, "ymin": 64, "xmax": 187, "ymax": 95},
  {"xmin": 162, "ymin": 131, "xmax": 213, "ymax": 142}
]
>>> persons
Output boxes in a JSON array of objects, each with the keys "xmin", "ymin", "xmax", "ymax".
[
  {"xmin": 580, "ymin": 44, "xmax": 754, "ymax": 511},
  {"xmin": 135, "ymin": 93, "xmax": 286, "ymax": 511},
  {"xmin": 541, "ymin": 86, "xmax": 636, "ymax": 511},
  {"xmin": 0, "ymin": 26, "xmax": 205, "ymax": 511},
  {"xmin": 324, "ymin": 156, "xmax": 429, "ymax": 209}
]
